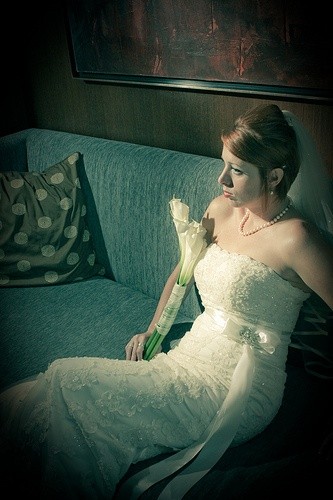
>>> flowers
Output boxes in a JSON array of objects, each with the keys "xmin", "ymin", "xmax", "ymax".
[{"xmin": 140, "ymin": 195, "xmax": 213, "ymax": 362}]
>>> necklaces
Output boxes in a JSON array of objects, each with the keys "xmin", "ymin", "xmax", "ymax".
[{"xmin": 239, "ymin": 195, "xmax": 293, "ymax": 236}]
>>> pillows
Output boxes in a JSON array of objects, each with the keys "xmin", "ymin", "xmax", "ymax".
[{"xmin": 0, "ymin": 151, "xmax": 116, "ymax": 289}]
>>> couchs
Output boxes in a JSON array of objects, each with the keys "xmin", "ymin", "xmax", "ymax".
[{"xmin": 0, "ymin": 126, "xmax": 332, "ymax": 500}]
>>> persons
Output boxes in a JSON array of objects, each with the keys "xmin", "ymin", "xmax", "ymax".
[{"xmin": 0, "ymin": 105, "xmax": 333, "ymax": 500}]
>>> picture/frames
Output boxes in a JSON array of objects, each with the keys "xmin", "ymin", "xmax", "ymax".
[{"xmin": 63, "ymin": 0, "xmax": 332, "ymax": 109}]
